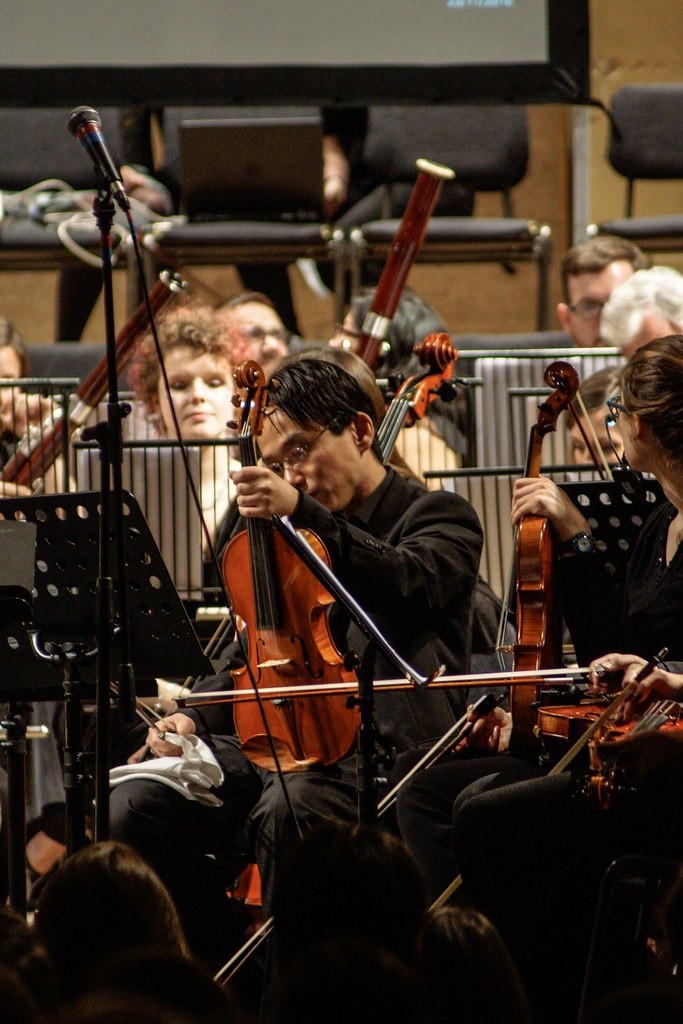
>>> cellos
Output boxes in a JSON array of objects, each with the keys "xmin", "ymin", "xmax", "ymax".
[{"xmin": 369, "ymin": 331, "xmax": 458, "ymax": 466}]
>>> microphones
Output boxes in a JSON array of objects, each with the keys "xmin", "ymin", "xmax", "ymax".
[{"xmin": 66, "ymin": 105, "xmax": 130, "ymax": 211}]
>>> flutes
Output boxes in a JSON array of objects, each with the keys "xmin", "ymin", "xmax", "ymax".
[
  {"xmin": 356, "ymin": 154, "xmax": 459, "ymax": 375},
  {"xmin": 0, "ymin": 267, "xmax": 189, "ymax": 491}
]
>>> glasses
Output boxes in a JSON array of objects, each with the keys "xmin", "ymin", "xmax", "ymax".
[
  {"xmin": 606, "ymin": 393, "xmax": 628, "ymax": 419},
  {"xmin": 267, "ymin": 412, "xmax": 345, "ymax": 477},
  {"xmin": 242, "ymin": 325, "xmax": 291, "ymax": 343},
  {"xmin": 333, "ymin": 322, "xmax": 362, "ymax": 337},
  {"xmin": 567, "ymin": 295, "xmax": 604, "ymax": 321}
]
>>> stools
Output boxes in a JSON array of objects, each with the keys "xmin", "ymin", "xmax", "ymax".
[{"xmin": 577, "ymin": 852, "xmax": 659, "ymax": 1024}]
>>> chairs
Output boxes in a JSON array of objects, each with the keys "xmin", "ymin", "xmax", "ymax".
[
  {"xmin": 349, "ymin": 105, "xmax": 552, "ymax": 330},
  {"xmin": 0, "ymin": 106, "xmax": 137, "ymax": 324},
  {"xmin": 598, "ymin": 84, "xmax": 683, "ymax": 252},
  {"xmin": 141, "ymin": 106, "xmax": 348, "ymax": 327}
]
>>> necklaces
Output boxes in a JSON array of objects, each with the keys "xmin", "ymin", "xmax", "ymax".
[{"xmin": 202, "ymin": 474, "xmax": 227, "ymax": 511}]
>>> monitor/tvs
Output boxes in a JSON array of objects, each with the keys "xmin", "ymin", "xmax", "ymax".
[{"xmin": 0, "ymin": 0, "xmax": 593, "ymax": 105}]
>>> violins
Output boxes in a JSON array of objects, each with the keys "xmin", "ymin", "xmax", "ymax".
[
  {"xmin": 219, "ymin": 357, "xmax": 362, "ymax": 774},
  {"xmin": 492, "ymin": 357, "xmax": 583, "ymax": 775},
  {"xmin": 537, "ymin": 695, "xmax": 683, "ymax": 812}
]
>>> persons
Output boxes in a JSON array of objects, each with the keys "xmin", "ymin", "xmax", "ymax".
[
  {"xmin": 282, "ymin": 819, "xmax": 530, "ymax": 1024},
  {"xmin": 329, "ymin": 285, "xmax": 459, "ymax": 489},
  {"xmin": 0, "ymin": 839, "xmax": 242, "ymax": 1024},
  {"xmin": 453, "ymin": 648, "xmax": 683, "ymax": 1024},
  {"xmin": 563, "ymin": 366, "xmax": 624, "ymax": 461},
  {"xmin": 0, "ymin": 320, "xmax": 84, "ymax": 518},
  {"xmin": 557, "ymin": 235, "xmax": 648, "ymax": 347},
  {"xmin": 109, "ymin": 357, "xmax": 485, "ymax": 1023},
  {"xmin": 128, "ymin": 305, "xmax": 255, "ymax": 539},
  {"xmin": 599, "ymin": 265, "xmax": 683, "ymax": 357},
  {"xmin": 394, "ymin": 336, "xmax": 683, "ymax": 898},
  {"xmin": 219, "ymin": 291, "xmax": 288, "ymax": 375}
]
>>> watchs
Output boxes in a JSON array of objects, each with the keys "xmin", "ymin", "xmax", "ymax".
[{"xmin": 558, "ymin": 530, "xmax": 598, "ymax": 556}]
